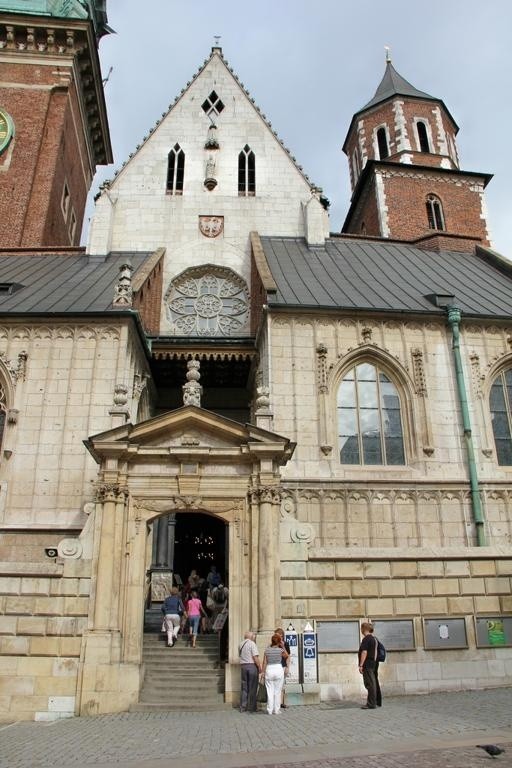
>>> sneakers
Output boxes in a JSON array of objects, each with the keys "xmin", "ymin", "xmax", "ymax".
[
  {"xmin": 268, "ymin": 710, "xmax": 282, "ymax": 715},
  {"xmin": 167, "ymin": 642, "xmax": 174, "ymax": 647},
  {"xmin": 173, "ymin": 634, "xmax": 178, "ymax": 639}
]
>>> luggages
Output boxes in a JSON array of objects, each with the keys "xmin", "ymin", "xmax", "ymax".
[{"xmin": 202, "ymin": 617, "xmax": 211, "ymax": 633}]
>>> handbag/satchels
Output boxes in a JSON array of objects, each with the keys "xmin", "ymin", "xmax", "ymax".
[
  {"xmin": 161, "ymin": 621, "xmax": 167, "ymax": 632},
  {"xmin": 256, "ymin": 676, "xmax": 268, "ymax": 703}
]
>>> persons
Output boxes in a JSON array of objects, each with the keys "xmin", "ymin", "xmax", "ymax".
[
  {"xmin": 160, "ymin": 565, "xmax": 229, "ymax": 647},
  {"xmin": 358, "ymin": 623, "xmax": 382, "ymax": 709},
  {"xmin": 238, "ymin": 628, "xmax": 290, "ymax": 715}
]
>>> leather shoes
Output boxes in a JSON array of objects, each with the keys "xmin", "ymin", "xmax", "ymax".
[
  {"xmin": 241, "ymin": 708, "xmax": 259, "ymax": 713},
  {"xmin": 361, "ymin": 702, "xmax": 381, "ymax": 709}
]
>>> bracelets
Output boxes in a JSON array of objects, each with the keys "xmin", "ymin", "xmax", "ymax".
[{"xmin": 360, "ymin": 666, "xmax": 363, "ymax": 667}]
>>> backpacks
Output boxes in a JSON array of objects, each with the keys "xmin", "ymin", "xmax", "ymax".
[
  {"xmin": 216, "ymin": 586, "xmax": 225, "ymax": 602},
  {"xmin": 373, "ymin": 636, "xmax": 386, "ymax": 662},
  {"xmin": 211, "ymin": 571, "xmax": 221, "ymax": 586}
]
